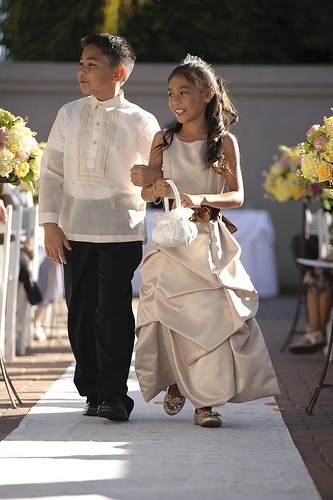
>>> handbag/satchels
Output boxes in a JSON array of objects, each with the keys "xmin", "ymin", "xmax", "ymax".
[{"xmin": 152, "ymin": 180, "xmax": 198, "ymax": 248}]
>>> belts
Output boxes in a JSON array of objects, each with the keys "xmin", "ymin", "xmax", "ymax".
[{"xmin": 163, "ymin": 198, "xmax": 221, "ymax": 222}]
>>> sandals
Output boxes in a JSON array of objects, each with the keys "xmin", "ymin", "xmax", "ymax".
[{"xmin": 288, "ymin": 330, "xmax": 328, "ymax": 354}]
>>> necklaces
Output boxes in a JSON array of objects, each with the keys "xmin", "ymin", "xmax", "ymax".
[{"xmin": 184, "ymin": 134, "xmax": 207, "ymax": 163}]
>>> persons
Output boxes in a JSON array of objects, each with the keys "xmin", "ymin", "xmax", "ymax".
[
  {"xmin": 141, "ymin": 61, "xmax": 280, "ymax": 427},
  {"xmin": 0, "ymin": 185, "xmax": 333, "ymax": 353},
  {"xmin": 38, "ymin": 33, "xmax": 164, "ymax": 422}
]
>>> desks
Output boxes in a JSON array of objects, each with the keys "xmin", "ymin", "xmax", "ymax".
[
  {"xmin": 131, "ymin": 208, "xmax": 278, "ymax": 297},
  {"xmin": 280, "ymin": 258, "xmax": 333, "ymax": 353}
]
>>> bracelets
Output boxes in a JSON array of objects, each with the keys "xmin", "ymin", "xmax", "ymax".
[{"xmin": 151, "ymin": 183, "xmax": 162, "ymax": 205}]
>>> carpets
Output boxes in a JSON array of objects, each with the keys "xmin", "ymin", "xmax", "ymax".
[{"xmin": 0, "ymin": 310, "xmax": 323, "ymax": 500}]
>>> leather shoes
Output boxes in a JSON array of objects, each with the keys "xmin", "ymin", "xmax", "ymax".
[
  {"xmin": 83, "ymin": 400, "xmax": 97, "ymax": 416},
  {"xmin": 97, "ymin": 399, "xmax": 129, "ymax": 421}
]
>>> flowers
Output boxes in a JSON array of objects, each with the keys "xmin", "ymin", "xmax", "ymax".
[
  {"xmin": 262, "ymin": 116, "xmax": 333, "ymax": 210},
  {"xmin": 0, "ymin": 107, "xmax": 47, "ymax": 196}
]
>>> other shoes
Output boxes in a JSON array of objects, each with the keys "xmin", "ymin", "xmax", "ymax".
[
  {"xmin": 194, "ymin": 408, "xmax": 222, "ymax": 427},
  {"xmin": 33, "ymin": 326, "xmax": 47, "ymax": 343},
  {"xmin": 163, "ymin": 386, "xmax": 186, "ymax": 415}
]
